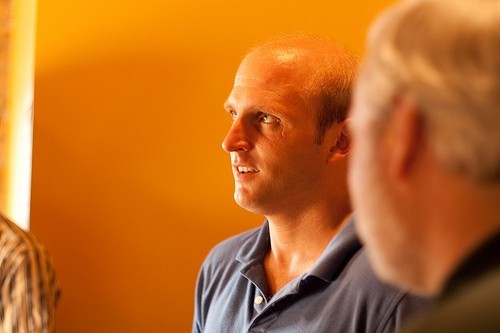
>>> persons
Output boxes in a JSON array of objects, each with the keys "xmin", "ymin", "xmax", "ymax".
[
  {"xmin": 345, "ymin": 1, "xmax": 500, "ymax": 333},
  {"xmin": 0, "ymin": 214, "xmax": 64, "ymax": 333},
  {"xmin": 189, "ymin": 37, "xmax": 412, "ymax": 333}
]
